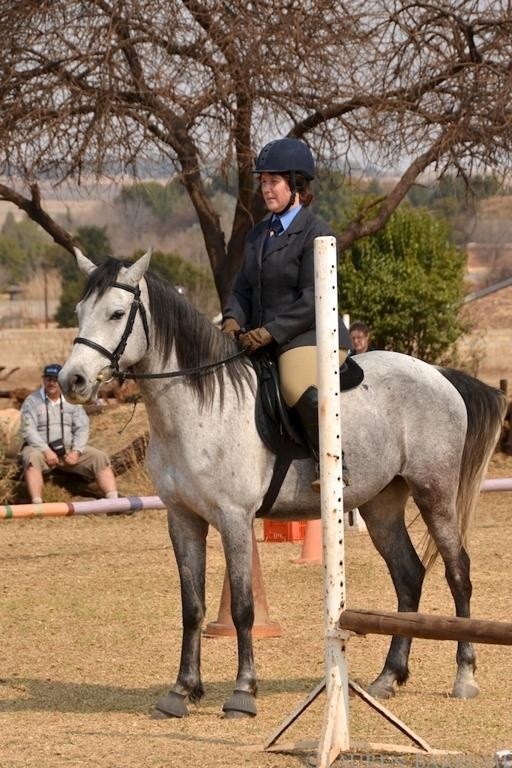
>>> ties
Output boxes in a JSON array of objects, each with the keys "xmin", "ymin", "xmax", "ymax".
[{"xmin": 264, "ymin": 220, "xmax": 284, "ymax": 253}]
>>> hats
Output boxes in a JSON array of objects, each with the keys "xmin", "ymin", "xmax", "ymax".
[{"xmin": 40, "ymin": 364, "xmax": 63, "ymax": 378}]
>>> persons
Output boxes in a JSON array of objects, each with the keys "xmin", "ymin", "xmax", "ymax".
[
  {"xmin": 219, "ymin": 138, "xmax": 356, "ymax": 491},
  {"xmin": 346, "ymin": 318, "xmax": 373, "ymax": 356},
  {"xmin": 19, "ymin": 365, "xmax": 120, "ymax": 502}
]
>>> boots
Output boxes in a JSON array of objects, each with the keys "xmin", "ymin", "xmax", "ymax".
[{"xmin": 287, "ymin": 384, "xmax": 351, "ymax": 493}]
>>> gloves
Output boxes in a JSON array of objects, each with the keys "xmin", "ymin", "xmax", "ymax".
[
  {"xmin": 238, "ymin": 327, "xmax": 273, "ymax": 358},
  {"xmin": 220, "ymin": 318, "xmax": 241, "ymax": 343}
]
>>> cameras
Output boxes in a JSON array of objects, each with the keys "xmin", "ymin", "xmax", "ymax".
[{"xmin": 50, "ymin": 439, "xmax": 67, "ymax": 457}]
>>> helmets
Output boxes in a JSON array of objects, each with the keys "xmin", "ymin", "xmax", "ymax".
[{"xmin": 250, "ymin": 139, "xmax": 315, "ymax": 180}]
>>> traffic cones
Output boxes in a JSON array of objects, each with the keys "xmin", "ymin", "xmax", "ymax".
[
  {"xmin": 288, "ymin": 517, "xmax": 323, "ymax": 566},
  {"xmin": 201, "ymin": 517, "xmax": 283, "ymax": 639}
]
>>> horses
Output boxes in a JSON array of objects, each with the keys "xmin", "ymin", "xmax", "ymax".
[{"xmin": 56, "ymin": 246, "xmax": 510, "ymax": 720}]
